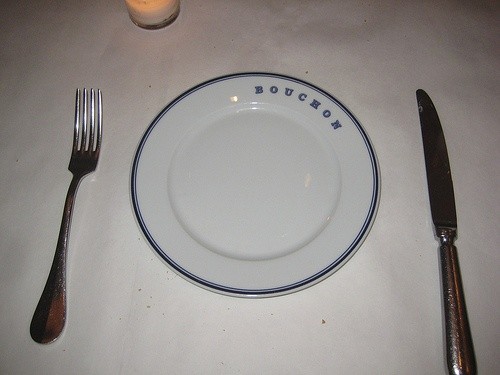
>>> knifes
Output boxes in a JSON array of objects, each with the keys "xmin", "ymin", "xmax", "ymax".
[{"xmin": 416, "ymin": 89, "xmax": 476, "ymax": 375}]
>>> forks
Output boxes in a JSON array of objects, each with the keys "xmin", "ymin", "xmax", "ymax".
[{"xmin": 30, "ymin": 88, "xmax": 104, "ymax": 343}]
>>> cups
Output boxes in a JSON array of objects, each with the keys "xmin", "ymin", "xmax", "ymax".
[{"xmin": 126, "ymin": 1, "xmax": 180, "ymax": 31}]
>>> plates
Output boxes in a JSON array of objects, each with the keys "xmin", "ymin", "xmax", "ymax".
[{"xmin": 131, "ymin": 73, "xmax": 381, "ymax": 298}]
